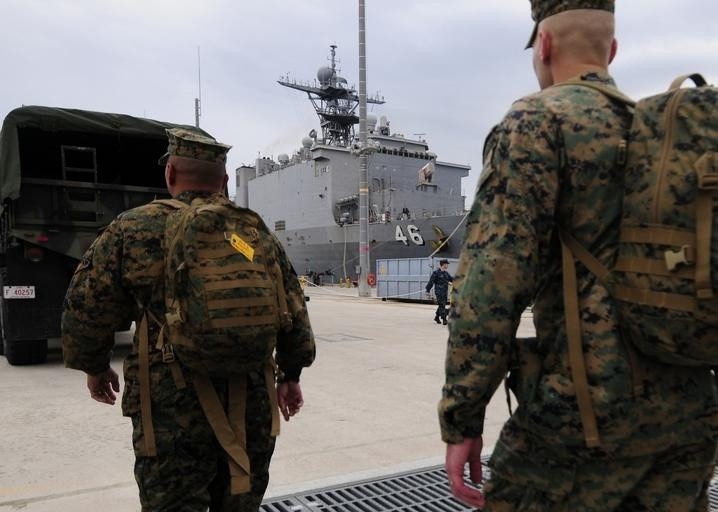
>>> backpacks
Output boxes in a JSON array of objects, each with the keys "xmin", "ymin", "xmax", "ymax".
[
  {"xmin": 146, "ymin": 194, "xmax": 285, "ymax": 380},
  {"xmin": 549, "ymin": 73, "xmax": 718, "ymax": 369}
]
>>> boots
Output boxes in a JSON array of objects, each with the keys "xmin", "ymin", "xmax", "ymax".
[
  {"xmin": 434, "ymin": 315, "xmax": 441, "ymax": 325},
  {"xmin": 443, "ymin": 319, "xmax": 447, "ymax": 325}
]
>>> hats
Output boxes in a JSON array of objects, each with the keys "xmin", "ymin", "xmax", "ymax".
[
  {"xmin": 440, "ymin": 258, "xmax": 450, "ymax": 264},
  {"xmin": 158, "ymin": 125, "xmax": 232, "ymax": 165},
  {"xmin": 523, "ymin": 0, "xmax": 615, "ymax": 50}
]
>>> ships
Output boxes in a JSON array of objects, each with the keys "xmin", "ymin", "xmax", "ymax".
[{"xmin": 236, "ymin": 44, "xmax": 472, "ymax": 284}]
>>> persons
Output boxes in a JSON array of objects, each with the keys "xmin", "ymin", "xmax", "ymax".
[
  {"xmin": 436, "ymin": 0, "xmax": 718, "ymax": 512},
  {"xmin": 59, "ymin": 126, "xmax": 316, "ymax": 512},
  {"xmin": 425, "ymin": 258, "xmax": 455, "ymax": 325}
]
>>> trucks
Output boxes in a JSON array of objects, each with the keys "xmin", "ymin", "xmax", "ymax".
[{"xmin": 0, "ymin": 105, "xmax": 229, "ymax": 366}]
